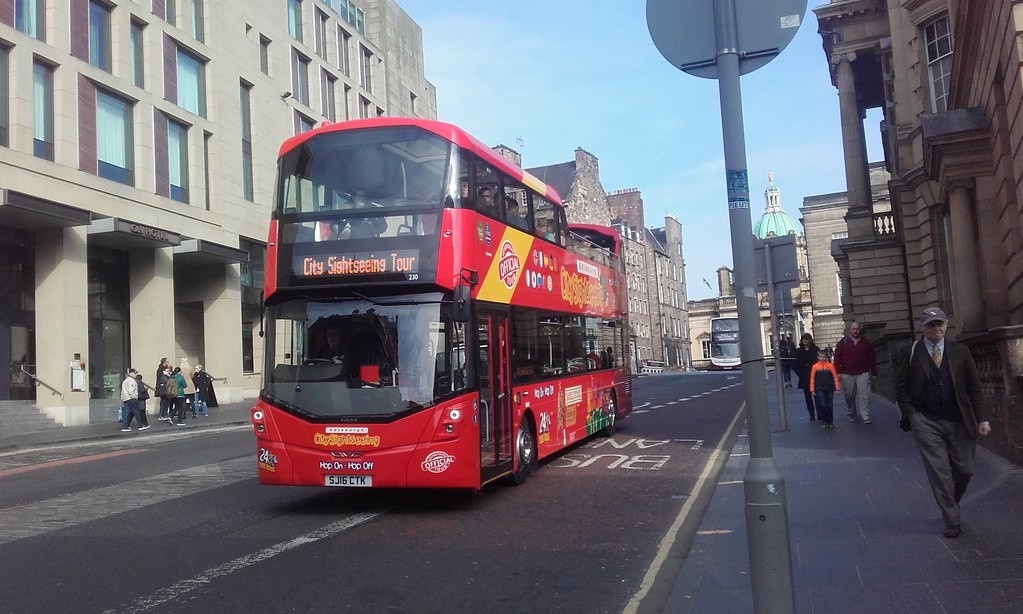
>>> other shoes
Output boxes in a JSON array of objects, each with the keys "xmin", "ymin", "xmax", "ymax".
[
  {"xmin": 327, "ymin": 225, "xmax": 338, "ymax": 240},
  {"xmin": 821, "ymin": 422, "xmax": 826, "ymax": 427},
  {"xmin": 828, "ymin": 424, "xmax": 834, "ymax": 428},
  {"xmin": 848, "ymin": 415, "xmax": 856, "ymax": 421},
  {"xmin": 864, "ymin": 419, "xmax": 871, "ymax": 423},
  {"xmin": 943, "ymin": 525, "xmax": 960, "ymax": 537},
  {"xmin": 340, "ymin": 228, "xmax": 351, "ymax": 239}
]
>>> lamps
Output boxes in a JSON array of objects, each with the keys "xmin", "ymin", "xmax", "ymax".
[{"xmin": 281, "ymin": 91, "xmax": 291, "ymax": 98}]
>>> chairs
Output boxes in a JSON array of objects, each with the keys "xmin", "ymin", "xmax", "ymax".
[{"xmin": 541, "ymin": 357, "xmax": 596, "ymax": 375}]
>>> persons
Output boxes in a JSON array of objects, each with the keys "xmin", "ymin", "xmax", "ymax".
[
  {"xmin": 810, "ymin": 349, "xmax": 840, "ymax": 429},
  {"xmin": 790, "ymin": 333, "xmax": 821, "ymax": 423},
  {"xmin": 833, "ymin": 321, "xmax": 878, "ymax": 425},
  {"xmin": 304, "ymin": 325, "xmax": 352, "ymax": 367},
  {"xmin": 599, "ymin": 346, "xmax": 613, "ymax": 368},
  {"xmin": 454, "ymin": 175, "xmax": 592, "ymax": 258},
  {"xmin": 894, "ymin": 307, "xmax": 992, "ymax": 539},
  {"xmin": 824, "ymin": 343, "xmax": 833, "ymax": 364},
  {"xmin": 120, "ymin": 358, "xmax": 210, "ymax": 432},
  {"xmin": 456, "ymin": 349, "xmax": 491, "ymax": 409},
  {"xmin": 773, "ymin": 336, "xmax": 797, "ymax": 390}
]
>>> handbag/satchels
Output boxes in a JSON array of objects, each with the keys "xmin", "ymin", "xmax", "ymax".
[
  {"xmin": 896, "ymin": 400, "xmax": 911, "ymax": 432},
  {"xmin": 117, "ymin": 406, "xmax": 123, "ymax": 422}
]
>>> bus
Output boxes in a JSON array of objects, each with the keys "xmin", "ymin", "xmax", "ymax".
[
  {"xmin": 709, "ymin": 316, "xmax": 742, "ymax": 370},
  {"xmin": 251, "ymin": 117, "xmax": 634, "ymax": 491}
]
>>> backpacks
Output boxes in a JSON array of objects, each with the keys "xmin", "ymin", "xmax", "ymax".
[{"xmin": 166, "ymin": 379, "xmax": 177, "ymax": 398}]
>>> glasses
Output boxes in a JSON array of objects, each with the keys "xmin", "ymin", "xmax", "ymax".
[{"xmin": 359, "ymin": 194, "xmax": 366, "ymax": 197}]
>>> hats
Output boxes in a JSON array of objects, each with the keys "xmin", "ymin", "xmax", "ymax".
[
  {"xmin": 921, "ymin": 307, "xmax": 946, "ymax": 324},
  {"xmin": 129, "ymin": 369, "xmax": 139, "ymax": 373}
]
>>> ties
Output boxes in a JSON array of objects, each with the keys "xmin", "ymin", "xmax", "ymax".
[{"xmin": 932, "ymin": 345, "xmax": 941, "ymax": 366}]
